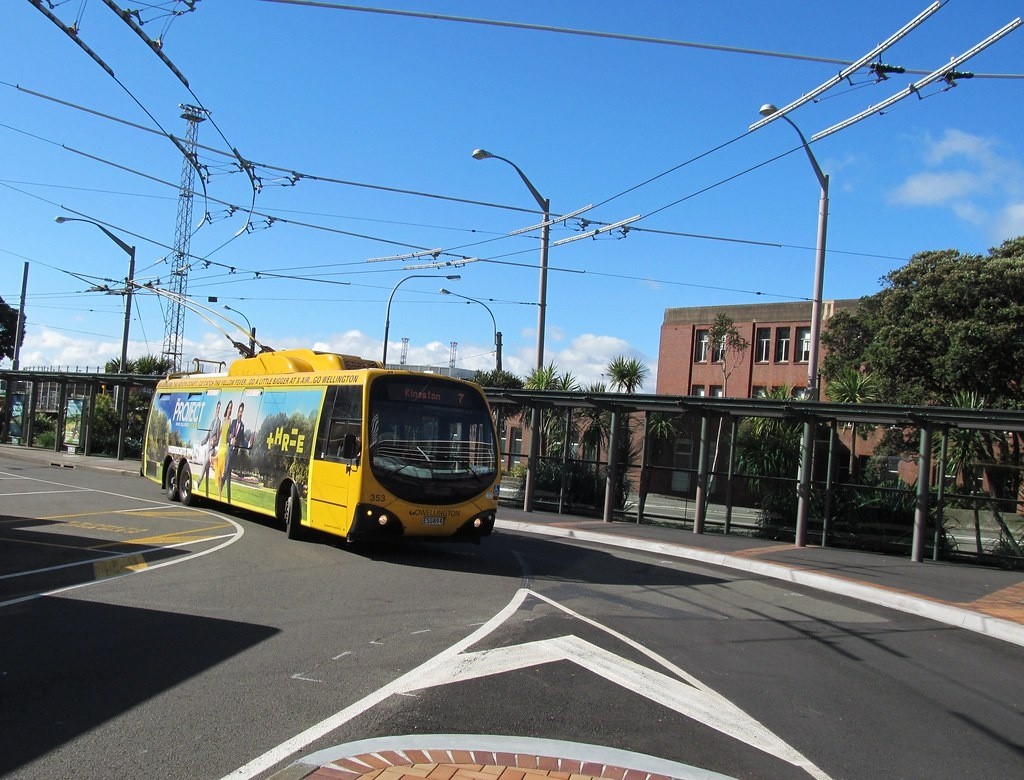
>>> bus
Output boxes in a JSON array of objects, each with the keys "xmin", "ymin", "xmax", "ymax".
[{"xmin": 141, "ymin": 349, "xmax": 503, "ymax": 546}]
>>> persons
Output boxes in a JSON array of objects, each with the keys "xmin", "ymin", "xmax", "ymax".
[{"xmin": 194, "ymin": 400, "xmax": 254, "ymax": 504}]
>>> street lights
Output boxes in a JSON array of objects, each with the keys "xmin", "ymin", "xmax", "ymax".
[
  {"xmin": 223, "ymin": 305, "xmax": 256, "ymax": 356},
  {"xmin": 757, "ymin": 103, "xmax": 830, "ymax": 547},
  {"xmin": 472, "ymin": 147, "xmax": 550, "ymax": 512},
  {"xmin": 55, "ymin": 215, "xmax": 136, "ymax": 460},
  {"xmin": 439, "ymin": 286, "xmax": 503, "ymax": 438},
  {"xmin": 382, "ymin": 274, "xmax": 461, "ymax": 368}
]
